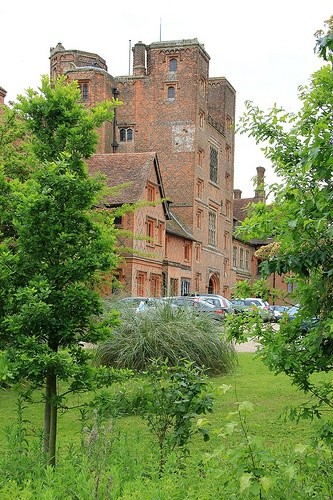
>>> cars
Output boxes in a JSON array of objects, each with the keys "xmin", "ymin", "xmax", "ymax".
[{"xmin": 114, "ymin": 294, "xmax": 317, "ymax": 331}]
